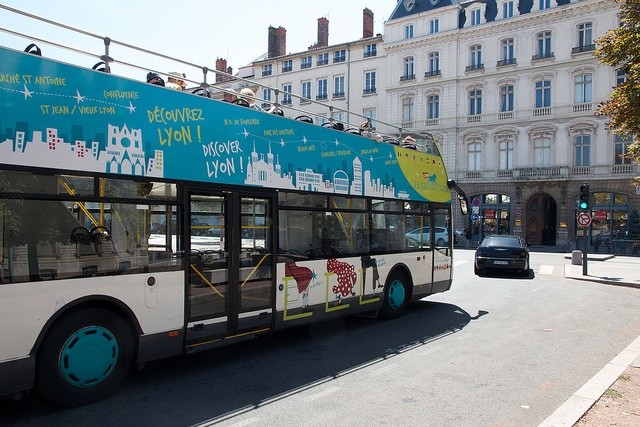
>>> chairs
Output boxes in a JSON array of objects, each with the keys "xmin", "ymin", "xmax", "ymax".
[
  {"xmin": 383, "ymin": 138, "xmax": 400, "ymax": 144},
  {"xmin": 92, "ymin": 61, "xmax": 111, "ymax": 74},
  {"xmin": 191, "ymin": 87, "xmax": 211, "ymax": 99},
  {"xmin": 159, "ymin": 243, "xmax": 387, "ymax": 284},
  {"xmin": 230, "ymin": 98, "xmax": 249, "ymax": 108},
  {"xmin": 343, "ymin": 127, "xmax": 361, "ymax": 135},
  {"xmin": 401, "ymin": 143, "xmax": 417, "ymax": 150},
  {"xmin": 70, "ymin": 226, "xmax": 98, "ymax": 275},
  {"xmin": 294, "ymin": 114, "xmax": 313, "ymax": 124},
  {"xmin": 320, "ymin": 122, "xmax": 340, "ymax": 131},
  {"xmin": 23, "ymin": 42, "xmax": 42, "ymax": 56},
  {"xmin": 265, "ymin": 106, "xmax": 284, "ymax": 116},
  {"xmin": 89, "ymin": 226, "xmax": 119, "ymax": 274}
]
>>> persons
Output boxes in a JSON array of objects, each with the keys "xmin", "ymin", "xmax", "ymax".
[
  {"xmin": 165, "ymin": 71, "xmax": 187, "ymax": 93},
  {"xmin": 405, "ymin": 135, "xmax": 428, "ymax": 153},
  {"xmin": 333, "ymin": 122, "xmax": 345, "ymax": 131},
  {"xmin": 146, "ymin": 72, "xmax": 160, "ymax": 84},
  {"xmin": 237, "ymin": 88, "xmax": 282, "ymax": 114},
  {"xmin": 221, "ymin": 88, "xmax": 237, "ymax": 103},
  {"xmin": 362, "ymin": 122, "xmax": 376, "ymax": 131}
]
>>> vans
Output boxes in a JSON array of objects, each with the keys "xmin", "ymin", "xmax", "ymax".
[{"xmin": 406, "ymin": 225, "xmax": 449, "ymax": 246}]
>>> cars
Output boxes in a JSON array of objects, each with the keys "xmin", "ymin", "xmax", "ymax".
[{"xmin": 474, "ymin": 233, "xmax": 529, "ymax": 275}]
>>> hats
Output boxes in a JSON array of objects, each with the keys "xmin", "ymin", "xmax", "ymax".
[
  {"xmin": 147, "ymin": 72, "xmax": 159, "ymax": 81},
  {"xmin": 223, "ymin": 88, "xmax": 237, "ymax": 98},
  {"xmin": 361, "ymin": 122, "xmax": 368, "ymax": 127},
  {"xmin": 405, "ymin": 136, "xmax": 415, "ymax": 141},
  {"xmin": 168, "ymin": 72, "xmax": 187, "ymax": 87}
]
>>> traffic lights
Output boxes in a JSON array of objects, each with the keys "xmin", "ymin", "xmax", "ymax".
[{"xmin": 579, "ymin": 183, "xmax": 590, "ymax": 211}]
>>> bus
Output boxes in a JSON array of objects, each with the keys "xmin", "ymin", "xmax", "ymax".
[{"xmin": 0, "ymin": 3, "xmax": 468, "ymax": 408}]
